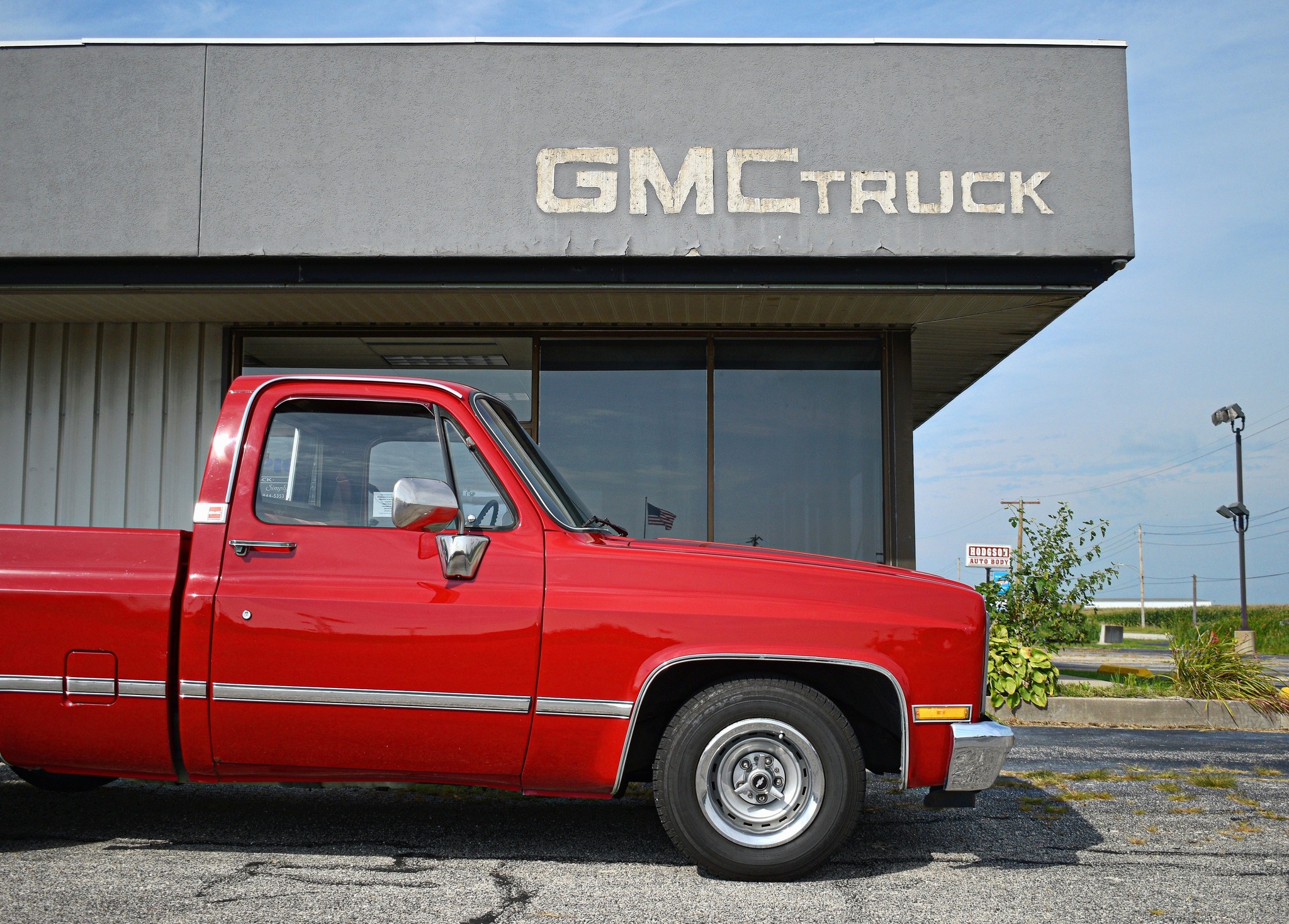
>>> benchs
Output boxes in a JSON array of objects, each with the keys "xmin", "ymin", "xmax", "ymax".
[{"xmin": 258, "ymin": 512, "xmax": 348, "ymax": 526}]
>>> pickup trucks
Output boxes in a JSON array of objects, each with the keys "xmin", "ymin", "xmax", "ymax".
[{"xmin": 0, "ymin": 374, "xmax": 1014, "ymax": 881}]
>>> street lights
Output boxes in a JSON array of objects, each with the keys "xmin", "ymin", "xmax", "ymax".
[
  {"xmin": 1118, "ymin": 564, "xmax": 1145, "ymax": 629},
  {"xmin": 1211, "ymin": 403, "xmax": 1256, "ymax": 655}
]
